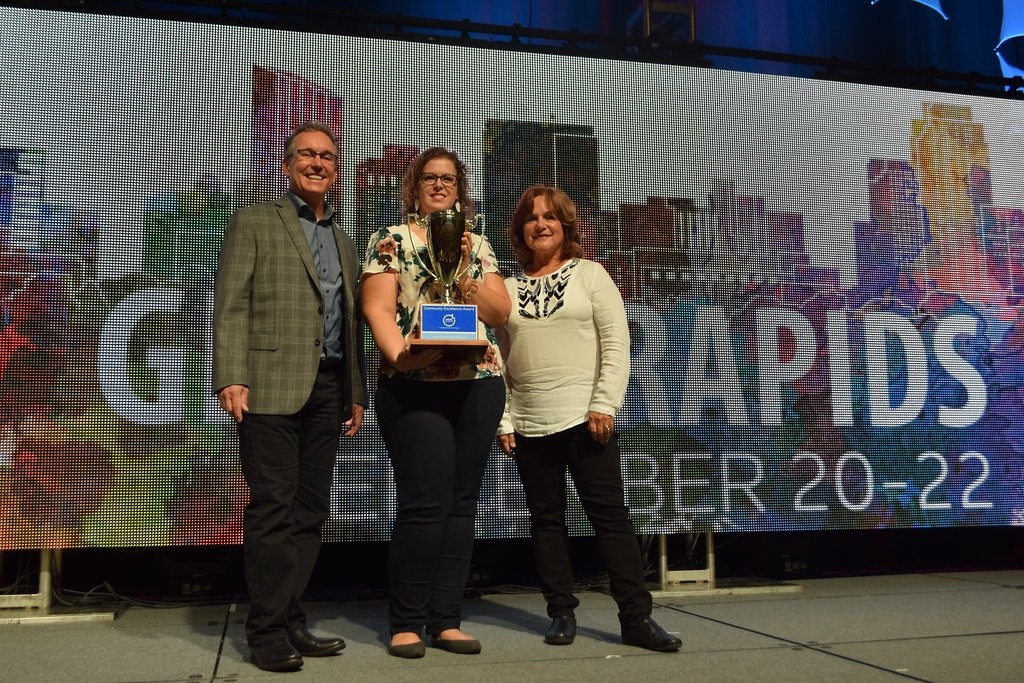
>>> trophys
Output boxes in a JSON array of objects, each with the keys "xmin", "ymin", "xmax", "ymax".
[{"xmin": 407, "ymin": 209, "xmax": 489, "ymax": 366}]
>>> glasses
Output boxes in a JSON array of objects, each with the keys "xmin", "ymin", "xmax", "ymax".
[
  {"xmin": 288, "ymin": 149, "xmax": 338, "ymax": 164},
  {"xmin": 419, "ymin": 172, "xmax": 461, "ymax": 187}
]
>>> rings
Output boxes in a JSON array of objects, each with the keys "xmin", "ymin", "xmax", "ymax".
[{"xmin": 605, "ymin": 425, "xmax": 609, "ymax": 428}]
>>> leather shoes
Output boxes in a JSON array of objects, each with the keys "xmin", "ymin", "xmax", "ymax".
[
  {"xmin": 544, "ymin": 615, "xmax": 577, "ymax": 645},
  {"xmin": 249, "ymin": 637, "xmax": 304, "ymax": 672},
  {"xmin": 620, "ymin": 616, "xmax": 683, "ymax": 651},
  {"xmin": 290, "ymin": 627, "xmax": 347, "ymax": 656}
]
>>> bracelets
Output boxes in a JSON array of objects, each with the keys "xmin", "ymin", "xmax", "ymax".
[{"xmin": 462, "ymin": 279, "xmax": 479, "ymax": 300}]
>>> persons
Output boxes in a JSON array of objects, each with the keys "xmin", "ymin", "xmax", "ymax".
[
  {"xmin": 486, "ymin": 187, "xmax": 682, "ymax": 651},
  {"xmin": 212, "ymin": 122, "xmax": 369, "ymax": 672},
  {"xmin": 361, "ymin": 146, "xmax": 512, "ymax": 657}
]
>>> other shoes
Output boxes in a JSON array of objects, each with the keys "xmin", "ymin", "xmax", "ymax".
[
  {"xmin": 389, "ymin": 630, "xmax": 427, "ymax": 658},
  {"xmin": 431, "ymin": 629, "xmax": 482, "ymax": 654}
]
